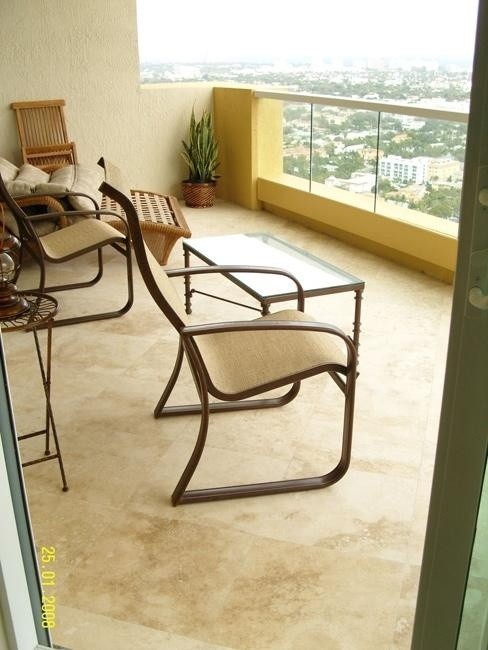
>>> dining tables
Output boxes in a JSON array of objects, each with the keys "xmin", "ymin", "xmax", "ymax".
[{"xmin": 178, "ymin": 99, "xmax": 223, "ymax": 209}]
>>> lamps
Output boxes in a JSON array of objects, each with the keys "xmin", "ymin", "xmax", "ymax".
[
  {"xmin": 0, "ymin": 174, "xmax": 134, "ymax": 332},
  {"xmin": 2, "ymin": 155, "xmax": 192, "ymax": 265},
  {"xmin": 95, "ymin": 155, "xmax": 358, "ymax": 507},
  {"xmin": 10, "ymin": 99, "xmax": 79, "ymax": 175}
]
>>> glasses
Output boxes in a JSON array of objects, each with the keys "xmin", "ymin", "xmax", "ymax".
[{"xmin": 0, "ymin": 233, "xmax": 30, "ymax": 320}]
